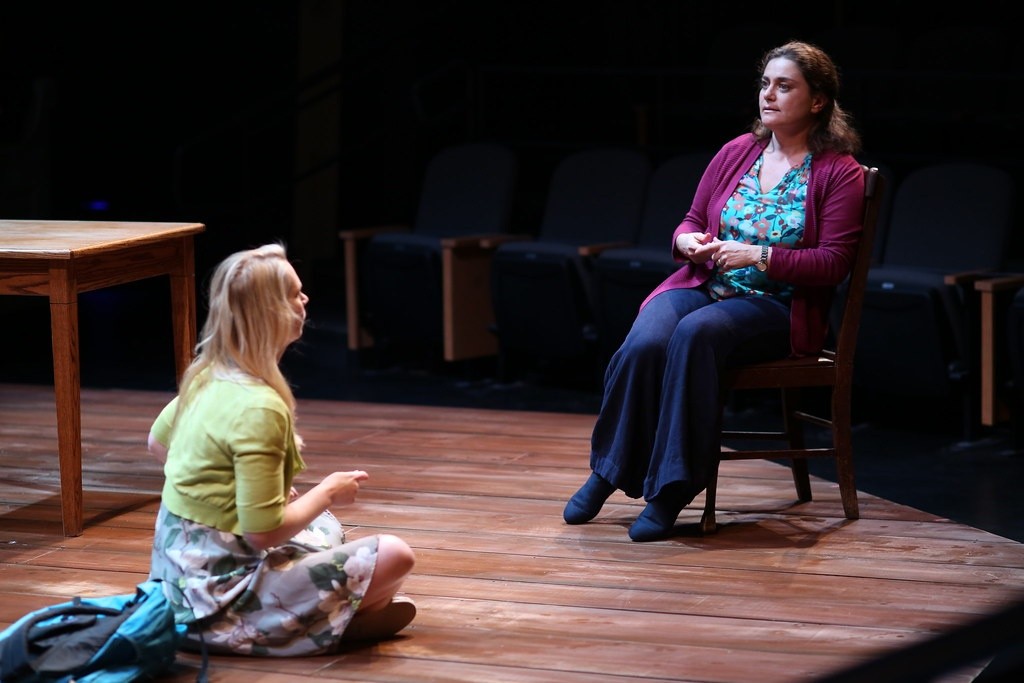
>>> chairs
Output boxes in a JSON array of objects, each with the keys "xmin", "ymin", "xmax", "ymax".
[
  {"xmin": 589, "ymin": 152, "xmax": 712, "ymax": 386},
  {"xmin": 338, "ymin": 140, "xmax": 531, "ymax": 364},
  {"xmin": 694, "ymin": 164, "xmax": 886, "ymax": 534},
  {"xmin": 482, "ymin": 147, "xmax": 666, "ymax": 383},
  {"xmin": 830, "ymin": 162, "xmax": 1007, "ymax": 440}
]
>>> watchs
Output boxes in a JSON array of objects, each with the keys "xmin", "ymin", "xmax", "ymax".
[{"xmin": 757, "ymin": 245, "xmax": 768, "ymax": 271}]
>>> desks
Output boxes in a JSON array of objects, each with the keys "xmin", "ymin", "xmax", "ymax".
[{"xmin": 0, "ymin": 220, "xmax": 206, "ymax": 539}]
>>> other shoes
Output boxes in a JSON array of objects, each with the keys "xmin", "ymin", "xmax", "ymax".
[
  {"xmin": 564, "ymin": 470, "xmax": 619, "ymax": 525},
  {"xmin": 627, "ymin": 496, "xmax": 691, "ymax": 543}
]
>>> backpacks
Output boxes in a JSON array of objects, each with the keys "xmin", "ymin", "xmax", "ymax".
[{"xmin": 0, "ymin": 580, "xmax": 188, "ymax": 683}]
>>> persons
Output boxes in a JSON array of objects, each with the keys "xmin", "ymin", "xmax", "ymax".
[
  {"xmin": 147, "ymin": 244, "xmax": 417, "ymax": 658},
  {"xmin": 563, "ymin": 42, "xmax": 867, "ymax": 541}
]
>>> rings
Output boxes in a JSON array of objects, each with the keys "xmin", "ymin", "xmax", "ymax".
[{"xmin": 718, "ymin": 259, "xmax": 723, "ymax": 265}]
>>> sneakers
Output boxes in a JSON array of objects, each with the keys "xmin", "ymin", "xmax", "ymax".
[{"xmin": 344, "ymin": 596, "xmax": 415, "ymax": 642}]
